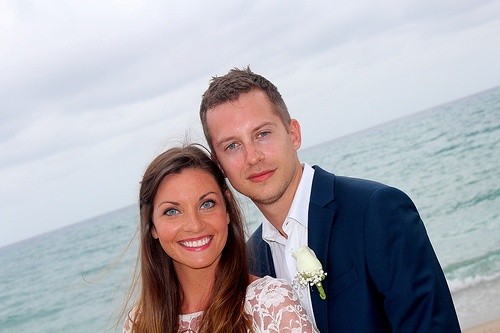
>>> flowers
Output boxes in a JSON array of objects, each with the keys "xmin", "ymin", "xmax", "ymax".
[{"xmin": 289, "ymin": 245, "xmax": 328, "ymax": 300}]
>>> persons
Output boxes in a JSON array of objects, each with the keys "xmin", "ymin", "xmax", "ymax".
[
  {"xmin": 199, "ymin": 65, "xmax": 462, "ymax": 333},
  {"xmin": 121, "ymin": 146, "xmax": 316, "ymax": 333}
]
